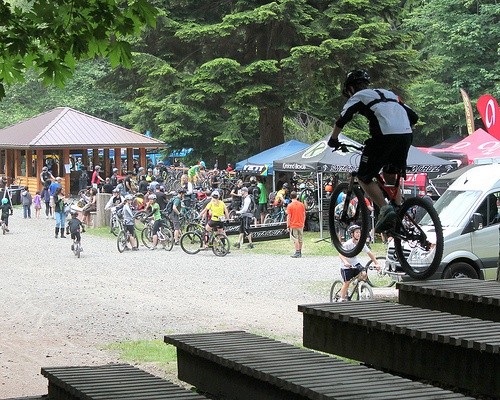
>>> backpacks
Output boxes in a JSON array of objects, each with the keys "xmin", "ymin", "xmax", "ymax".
[{"xmin": 253, "ymin": 188, "xmax": 259, "ymax": 202}]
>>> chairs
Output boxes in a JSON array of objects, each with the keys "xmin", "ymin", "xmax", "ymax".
[{"xmin": 476, "ymin": 193, "xmax": 500, "ymax": 226}]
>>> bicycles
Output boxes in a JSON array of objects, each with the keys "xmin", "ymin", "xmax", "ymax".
[
  {"xmin": 1, "ymin": 214, "xmax": 12, "ymax": 235},
  {"xmin": 66, "ymin": 230, "xmax": 86, "ymax": 258},
  {"xmin": 328, "ymin": 144, "xmax": 444, "ymax": 279},
  {"xmin": 364, "ymin": 256, "xmax": 400, "ymax": 288},
  {"xmin": 79, "ymin": 168, "xmax": 332, "ymax": 257},
  {"xmin": 330, "ymin": 266, "xmax": 376, "ymax": 303}
]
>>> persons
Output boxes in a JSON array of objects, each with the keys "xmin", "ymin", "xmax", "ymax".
[
  {"xmin": 334, "ymin": 191, "xmax": 374, "ymax": 244},
  {"xmin": 0, "ymin": 157, "xmax": 289, "ymax": 256},
  {"xmin": 327, "ymin": 69, "xmax": 420, "ymax": 234},
  {"xmin": 339, "ymin": 225, "xmax": 381, "ymax": 302},
  {"xmin": 285, "ymin": 191, "xmax": 306, "ymax": 258}
]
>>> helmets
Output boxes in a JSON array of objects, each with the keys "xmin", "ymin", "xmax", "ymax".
[
  {"xmin": 55, "ymin": 177, "xmax": 62, "ymax": 181},
  {"xmin": 342, "ymin": 70, "xmax": 371, "ymax": 93},
  {"xmin": 125, "ymin": 194, "xmax": 133, "ymax": 200},
  {"xmin": 347, "ymin": 224, "xmax": 360, "ymax": 234},
  {"xmin": 179, "ymin": 188, "xmax": 186, "ymax": 194},
  {"xmin": 148, "ymin": 194, "xmax": 157, "ymax": 200},
  {"xmin": 283, "ymin": 183, "xmax": 289, "ymax": 188},
  {"xmin": 42, "ymin": 167, "xmax": 47, "ymax": 171},
  {"xmin": 113, "ymin": 168, "xmax": 118, "ymax": 171},
  {"xmin": 112, "ymin": 188, "xmax": 120, "ymax": 193}
]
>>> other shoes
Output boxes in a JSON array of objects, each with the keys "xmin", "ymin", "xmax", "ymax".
[
  {"xmin": 290, "ymin": 253, "xmax": 302, "ymax": 258},
  {"xmin": 375, "ymin": 208, "xmax": 396, "ymax": 233},
  {"xmin": 55, "ymin": 235, "xmax": 66, "ymax": 238},
  {"xmin": 247, "ymin": 243, "xmax": 254, "ymax": 248},
  {"xmin": 124, "ymin": 247, "xmax": 139, "ymax": 251},
  {"xmin": 233, "ymin": 242, "xmax": 240, "ymax": 248},
  {"xmin": 174, "ymin": 240, "xmax": 180, "ymax": 245},
  {"xmin": 151, "ymin": 246, "xmax": 158, "ymax": 250}
]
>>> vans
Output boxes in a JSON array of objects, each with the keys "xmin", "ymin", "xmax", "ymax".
[{"xmin": 385, "ymin": 163, "xmax": 499, "ymax": 282}]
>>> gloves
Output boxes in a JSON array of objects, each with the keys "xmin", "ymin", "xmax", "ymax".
[{"xmin": 328, "ymin": 137, "xmax": 339, "ymax": 147}]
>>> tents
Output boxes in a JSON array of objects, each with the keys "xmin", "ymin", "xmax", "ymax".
[
  {"xmin": 405, "ymin": 126, "xmax": 500, "ymax": 201},
  {"xmin": 236, "ymin": 139, "xmax": 312, "ymax": 197},
  {"xmin": 272, "ymin": 130, "xmax": 376, "ymax": 245}
]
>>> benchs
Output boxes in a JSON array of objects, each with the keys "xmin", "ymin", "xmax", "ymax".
[
  {"xmin": 164, "ymin": 329, "xmax": 478, "ymax": 400},
  {"xmin": 70, "ymin": 205, "xmax": 97, "ymax": 228},
  {"xmin": 395, "ymin": 275, "xmax": 500, "ymax": 324},
  {"xmin": 295, "ymin": 298, "xmax": 500, "ymax": 400},
  {"xmin": 39, "ymin": 360, "xmax": 213, "ymax": 400}
]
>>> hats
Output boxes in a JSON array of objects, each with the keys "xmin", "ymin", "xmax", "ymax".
[
  {"xmin": 95, "ymin": 166, "xmax": 101, "ymax": 169},
  {"xmin": 211, "ymin": 191, "xmax": 220, "ymax": 199},
  {"xmin": 2, "ymin": 198, "xmax": 8, "ymax": 203},
  {"xmin": 200, "ymin": 161, "xmax": 205, "ymax": 167},
  {"xmin": 241, "ymin": 187, "xmax": 248, "ymax": 192},
  {"xmin": 250, "ymin": 176, "xmax": 257, "ymax": 184}
]
